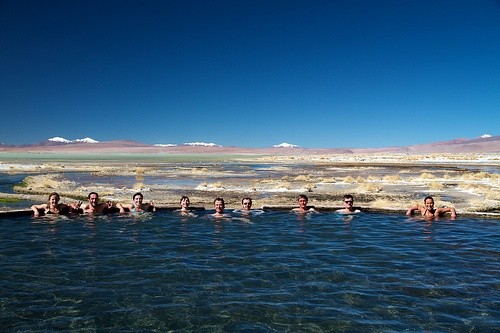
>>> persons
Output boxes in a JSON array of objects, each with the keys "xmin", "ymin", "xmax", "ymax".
[
  {"xmin": 214, "ymin": 198, "xmax": 225, "ymax": 214},
  {"xmin": 69, "ymin": 192, "xmax": 113, "ymax": 213},
  {"xmin": 291, "ymin": 194, "xmax": 315, "ymax": 211},
  {"xmin": 242, "ymin": 197, "xmax": 252, "ymax": 210},
  {"xmin": 180, "ymin": 196, "xmax": 190, "ymax": 210},
  {"xmin": 335, "ymin": 195, "xmax": 360, "ymax": 213},
  {"xmin": 31, "ymin": 192, "xmax": 68, "ymax": 216},
  {"xmin": 406, "ymin": 196, "xmax": 457, "ymax": 219},
  {"xmin": 116, "ymin": 193, "xmax": 156, "ymax": 212}
]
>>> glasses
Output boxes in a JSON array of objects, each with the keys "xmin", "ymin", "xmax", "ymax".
[
  {"xmin": 244, "ymin": 203, "xmax": 251, "ymax": 205},
  {"xmin": 345, "ymin": 201, "xmax": 353, "ymax": 204}
]
